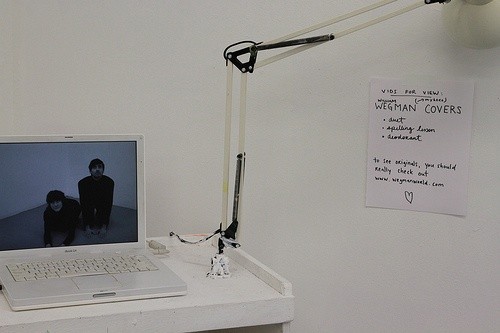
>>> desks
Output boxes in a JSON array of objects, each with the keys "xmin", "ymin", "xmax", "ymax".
[{"xmin": 0, "ymin": 231, "xmax": 297, "ymax": 333}]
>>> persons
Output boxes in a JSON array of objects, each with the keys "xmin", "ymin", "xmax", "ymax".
[
  {"xmin": 78, "ymin": 159, "xmax": 114, "ymax": 238},
  {"xmin": 44, "ymin": 190, "xmax": 81, "ymax": 247}
]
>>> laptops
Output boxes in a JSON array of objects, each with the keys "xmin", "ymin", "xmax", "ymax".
[{"xmin": 0, "ymin": 134, "xmax": 188, "ymax": 312}]
